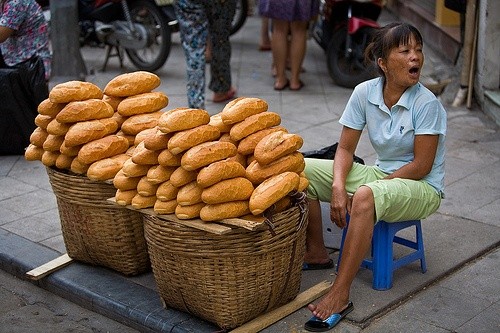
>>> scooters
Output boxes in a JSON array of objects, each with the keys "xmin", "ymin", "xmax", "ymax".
[
  {"xmin": 37, "ymin": 0, "xmax": 249, "ymax": 81},
  {"xmin": 313, "ymin": 0, "xmax": 382, "ymax": 88}
]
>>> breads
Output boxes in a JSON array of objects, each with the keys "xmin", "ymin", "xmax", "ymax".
[
  {"xmin": 114, "ymin": 98, "xmax": 310, "ymax": 222},
  {"xmin": 24, "ymin": 71, "xmax": 169, "ymax": 181}
]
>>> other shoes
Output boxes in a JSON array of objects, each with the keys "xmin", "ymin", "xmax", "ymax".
[
  {"xmin": 285, "ymin": 64, "xmax": 306, "ymax": 73},
  {"xmin": 212, "ymin": 87, "xmax": 235, "ymax": 103}
]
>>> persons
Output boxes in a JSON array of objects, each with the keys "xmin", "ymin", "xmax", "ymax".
[
  {"xmin": 258, "ymin": 0, "xmax": 320, "ymax": 92},
  {"xmin": 302, "ymin": 21, "xmax": 447, "ymax": 321},
  {"xmin": 173, "ymin": 0, "xmax": 237, "ymax": 111},
  {"xmin": 0, "ymin": 0, "xmax": 53, "ymax": 80}
]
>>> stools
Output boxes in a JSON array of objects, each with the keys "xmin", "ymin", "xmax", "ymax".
[{"xmin": 336, "ymin": 212, "xmax": 426, "ymax": 291}]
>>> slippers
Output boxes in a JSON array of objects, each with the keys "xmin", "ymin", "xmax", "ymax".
[
  {"xmin": 305, "ymin": 300, "xmax": 354, "ymax": 332},
  {"xmin": 274, "ymin": 78, "xmax": 290, "ymax": 90},
  {"xmin": 289, "ymin": 80, "xmax": 304, "ymax": 91},
  {"xmin": 302, "ymin": 258, "xmax": 335, "ymax": 270}
]
